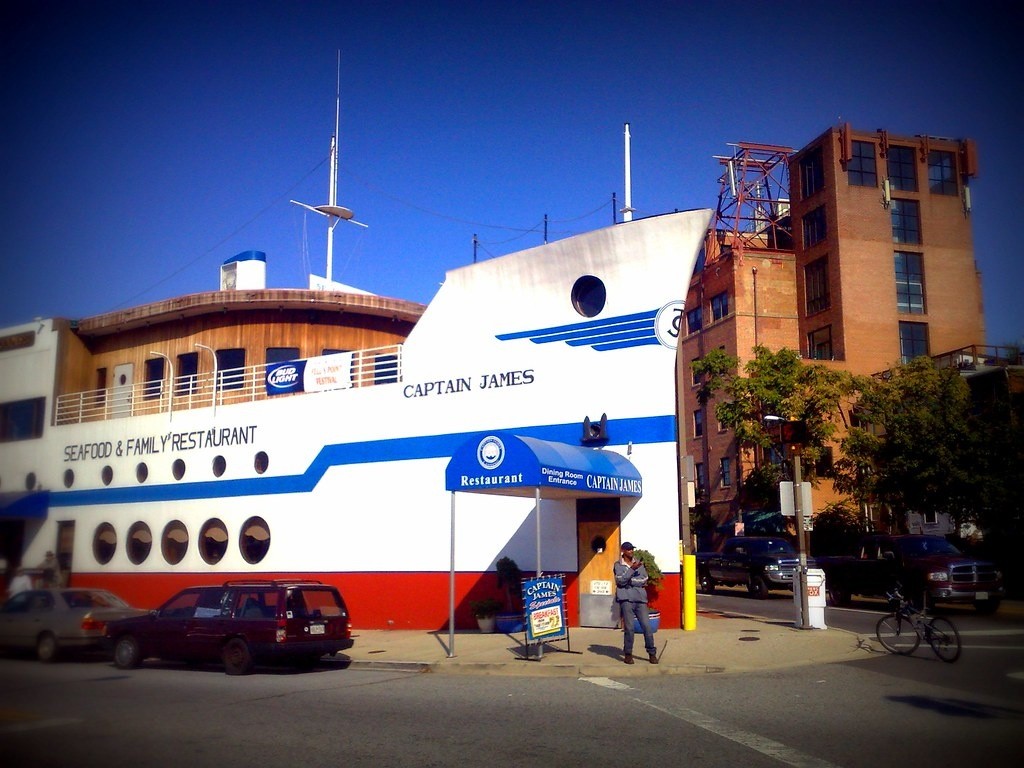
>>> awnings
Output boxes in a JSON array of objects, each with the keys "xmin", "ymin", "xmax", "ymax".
[{"xmin": 445, "ymin": 430, "xmax": 642, "ymax": 653}]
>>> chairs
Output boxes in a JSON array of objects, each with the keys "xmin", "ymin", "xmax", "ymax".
[
  {"xmin": 287, "ymin": 590, "xmax": 306, "ymax": 618},
  {"xmin": 244, "ymin": 598, "xmax": 265, "ymax": 619}
]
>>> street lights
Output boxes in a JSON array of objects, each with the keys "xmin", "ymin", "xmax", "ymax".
[{"xmin": 765, "ymin": 414, "xmax": 809, "ymax": 629}]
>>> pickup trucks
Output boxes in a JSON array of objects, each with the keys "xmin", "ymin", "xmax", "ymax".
[
  {"xmin": 693, "ymin": 535, "xmax": 816, "ymax": 596},
  {"xmin": 816, "ymin": 533, "xmax": 1005, "ymax": 617}
]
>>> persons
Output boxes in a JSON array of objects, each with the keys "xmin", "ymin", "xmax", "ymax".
[
  {"xmin": 8, "ymin": 566, "xmax": 32, "ymax": 600},
  {"xmin": 37, "ymin": 551, "xmax": 62, "ymax": 589},
  {"xmin": 613, "ymin": 542, "xmax": 658, "ymax": 664}
]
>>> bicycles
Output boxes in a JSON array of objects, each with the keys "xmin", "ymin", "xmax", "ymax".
[{"xmin": 875, "ymin": 587, "xmax": 962, "ymax": 664}]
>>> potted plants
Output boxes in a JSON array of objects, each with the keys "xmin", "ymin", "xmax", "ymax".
[
  {"xmin": 469, "ymin": 597, "xmax": 506, "ymax": 634},
  {"xmin": 495, "ymin": 557, "xmax": 526, "ymax": 633},
  {"xmin": 632, "ymin": 549, "xmax": 662, "ymax": 634}
]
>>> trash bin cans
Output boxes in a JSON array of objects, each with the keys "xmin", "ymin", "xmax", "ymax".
[{"xmin": 792, "ymin": 566, "xmax": 827, "ymax": 630}]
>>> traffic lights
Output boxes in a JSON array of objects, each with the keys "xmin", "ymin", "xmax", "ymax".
[{"xmin": 778, "ymin": 420, "xmax": 806, "ymax": 444}]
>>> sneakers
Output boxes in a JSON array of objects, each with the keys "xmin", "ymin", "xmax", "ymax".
[
  {"xmin": 649, "ymin": 655, "xmax": 659, "ymax": 663},
  {"xmin": 624, "ymin": 653, "xmax": 634, "ymax": 664}
]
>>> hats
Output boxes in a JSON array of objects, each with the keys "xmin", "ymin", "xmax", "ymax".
[{"xmin": 621, "ymin": 542, "xmax": 635, "ymax": 549}]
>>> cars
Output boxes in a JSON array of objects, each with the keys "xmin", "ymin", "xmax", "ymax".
[
  {"xmin": 0, "ymin": 588, "xmax": 154, "ymax": 661},
  {"xmin": 108, "ymin": 580, "xmax": 353, "ymax": 673},
  {"xmin": 955, "ymin": 523, "xmax": 984, "ymax": 540}
]
